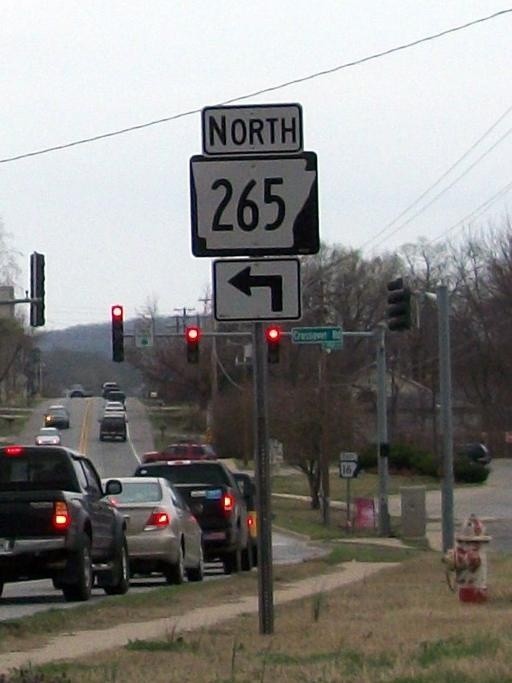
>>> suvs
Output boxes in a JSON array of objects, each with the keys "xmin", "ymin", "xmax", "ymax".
[
  {"xmin": 133, "ymin": 459, "xmax": 257, "ymax": 574},
  {"xmin": 141, "ymin": 444, "xmax": 218, "ymax": 464},
  {"xmin": 231, "ymin": 472, "xmax": 257, "ymax": 567},
  {"xmin": 0, "ymin": 446, "xmax": 131, "ymax": 601},
  {"xmin": 43, "ymin": 405, "xmax": 71, "ymax": 430},
  {"xmin": 97, "ymin": 382, "xmax": 129, "ymax": 441}
]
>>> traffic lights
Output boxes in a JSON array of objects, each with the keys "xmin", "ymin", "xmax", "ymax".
[
  {"xmin": 185, "ymin": 327, "xmax": 200, "ymax": 365},
  {"xmin": 386, "ymin": 276, "xmax": 413, "ymax": 331},
  {"xmin": 265, "ymin": 324, "xmax": 282, "ymax": 365},
  {"xmin": 111, "ymin": 304, "xmax": 124, "ymax": 361}
]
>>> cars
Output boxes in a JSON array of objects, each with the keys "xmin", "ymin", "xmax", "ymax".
[
  {"xmin": 101, "ymin": 477, "xmax": 205, "ymax": 585},
  {"xmin": 69, "ymin": 388, "xmax": 85, "ymax": 398},
  {"xmin": 34, "ymin": 426, "xmax": 62, "ymax": 446},
  {"xmin": 462, "ymin": 443, "xmax": 491, "ymax": 465}
]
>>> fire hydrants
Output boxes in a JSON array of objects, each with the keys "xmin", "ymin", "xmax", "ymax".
[{"xmin": 439, "ymin": 513, "xmax": 492, "ymax": 602}]
